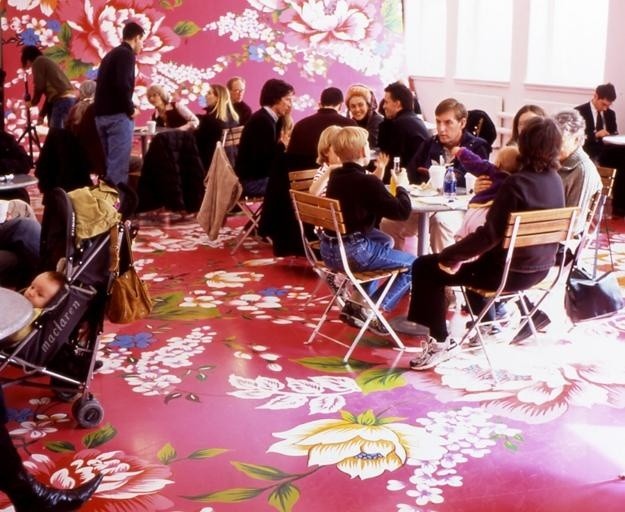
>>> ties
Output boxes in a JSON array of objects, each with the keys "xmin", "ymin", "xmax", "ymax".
[{"xmin": 597, "ymin": 111, "xmax": 603, "ymax": 131}]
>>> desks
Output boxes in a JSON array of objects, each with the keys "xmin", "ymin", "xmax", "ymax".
[{"xmin": 0, "ymin": 172, "xmax": 42, "ymax": 340}]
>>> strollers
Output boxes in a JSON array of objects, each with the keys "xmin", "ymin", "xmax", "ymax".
[{"xmin": 0, "ymin": 172, "xmax": 138, "ymax": 427}]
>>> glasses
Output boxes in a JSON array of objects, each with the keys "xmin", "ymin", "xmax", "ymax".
[{"xmin": 147, "ymin": 93, "xmax": 160, "ymax": 99}]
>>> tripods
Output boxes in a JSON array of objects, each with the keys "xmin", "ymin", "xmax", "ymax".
[{"xmin": 17, "ymin": 80, "xmax": 43, "ymax": 169}]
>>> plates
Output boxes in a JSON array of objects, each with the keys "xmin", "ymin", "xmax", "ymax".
[{"xmin": 409, "ymin": 188, "xmax": 440, "ymax": 197}]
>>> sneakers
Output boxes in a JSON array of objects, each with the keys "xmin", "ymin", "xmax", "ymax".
[
  {"xmin": 408, "ymin": 331, "xmax": 463, "ymax": 371},
  {"xmin": 462, "ymin": 308, "xmax": 509, "ymax": 338},
  {"xmin": 349, "ymin": 306, "xmax": 392, "ymax": 337},
  {"xmin": 339, "ymin": 303, "xmax": 356, "ymax": 326}
]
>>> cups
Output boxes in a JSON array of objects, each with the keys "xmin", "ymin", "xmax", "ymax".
[
  {"xmin": 465, "ymin": 177, "xmax": 475, "ymax": 196},
  {"xmin": 146, "ymin": 120, "xmax": 156, "ymax": 133},
  {"xmin": 428, "ymin": 166, "xmax": 445, "ymax": 189}
]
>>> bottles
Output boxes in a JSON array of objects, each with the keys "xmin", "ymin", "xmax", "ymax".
[
  {"xmin": 442, "ymin": 168, "xmax": 457, "ymax": 201},
  {"xmin": 390, "ymin": 157, "xmax": 403, "ymax": 195}
]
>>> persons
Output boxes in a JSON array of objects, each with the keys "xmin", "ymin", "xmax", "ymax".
[
  {"xmin": 193, "ymin": 83, "xmax": 238, "ymax": 170},
  {"xmin": 225, "ymin": 76, "xmax": 251, "ymax": 125},
  {"xmin": 92, "ymin": 21, "xmax": 147, "ymax": 221},
  {"xmin": 142, "ymin": 84, "xmax": 200, "ymax": 131},
  {"xmin": 19, "ymin": 45, "xmax": 75, "ymax": 132},
  {"xmin": 0, "ymin": 130, "xmax": 33, "ymax": 205},
  {"xmin": 569, "ymin": 84, "xmax": 625, "ymax": 222},
  {"xmin": 0, "ymin": 270, "xmax": 70, "ymax": 350},
  {"xmin": 308, "ymin": 124, "xmax": 419, "ymax": 335},
  {"xmin": 64, "ymin": 77, "xmax": 145, "ymax": 191},
  {"xmin": 0, "ymin": 385, "xmax": 105, "ymax": 512},
  {"xmin": 382, "ymin": 103, "xmax": 604, "ymax": 371},
  {"xmin": 0, "ymin": 199, "xmax": 46, "ymax": 275},
  {"xmin": 406, "ymin": 98, "xmax": 490, "ymax": 187},
  {"xmin": 235, "ymin": 78, "xmax": 294, "ymax": 241},
  {"xmin": 260, "ymin": 81, "xmax": 432, "ymax": 256}
]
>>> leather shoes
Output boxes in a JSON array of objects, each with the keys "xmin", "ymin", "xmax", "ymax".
[
  {"xmin": 510, "ymin": 309, "xmax": 551, "ymax": 344},
  {"xmin": 12, "ymin": 473, "xmax": 104, "ymax": 512}
]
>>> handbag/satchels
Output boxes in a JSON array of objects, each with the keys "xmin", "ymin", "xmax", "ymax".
[
  {"xmin": 107, "ymin": 268, "xmax": 153, "ymax": 324},
  {"xmin": 564, "ymin": 266, "xmax": 624, "ymax": 324}
]
